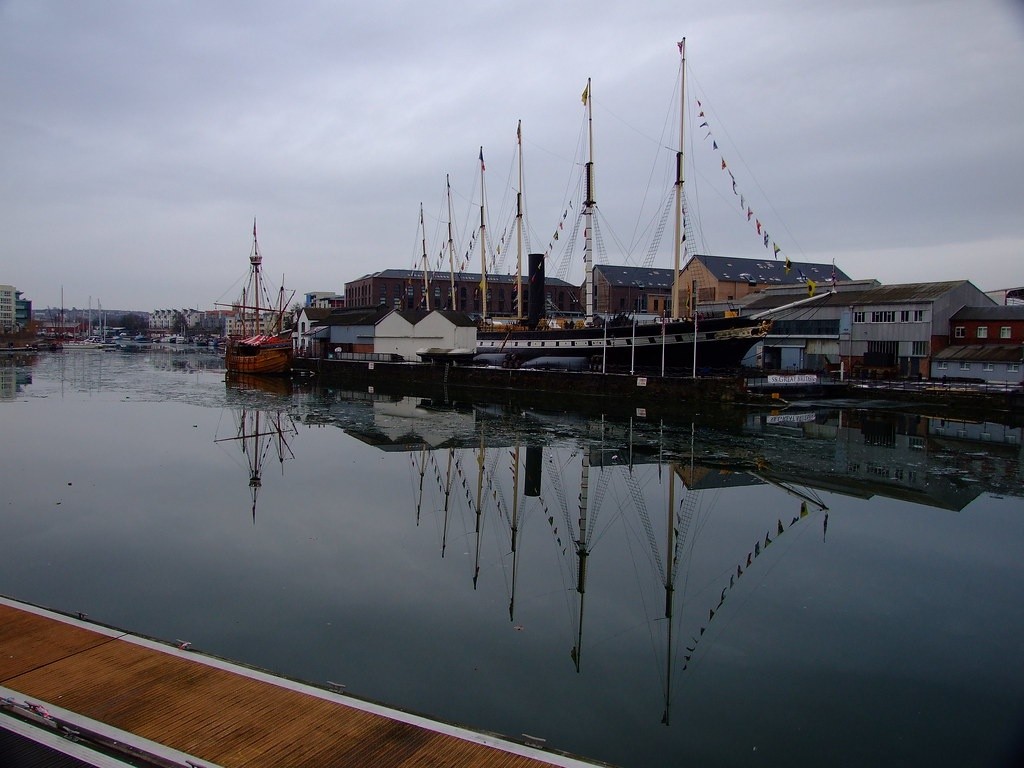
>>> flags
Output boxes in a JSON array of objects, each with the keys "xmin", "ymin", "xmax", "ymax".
[
  {"xmin": 785, "ymin": 257, "xmax": 792, "ymax": 276},
  {"xmin": 733, "ymin": 180, "xmax": 737, "ymax": 195},
  {"xmin": 682, "ymin": 208, "xmax": 689, "ymax": 262},
  {"xmin": 400, "ymin": 227, "xmax": 519, "ymax": 309},
  {"xmin": 696, "ymin": 101, "xmax": 727, "ymax": 170},
  {"xmin": 518, "ymin": 123, "xmax": 521, "ymax": 145},
  {"xmin": 773, "ymin": 243, "xmax": 780, "ymax": 259},
  {"xmin": 581, "ymin": 84, "xmax": 588, "ymax": 106},
  {"xmin": 747, "ymin": 206, "xmax": 753, "ymax": 221},
  {"xmin": 756, "ymin": 219, "xmax": 761, "ymax": 235},
  {"xmin": 741, "ymin": 195, "xmax": 745, "ymax": 209},
  {"xmin": 583, "ymin": 228, "xmax": 588, "ymax": 263},
  {"xmin": 411, "ymin": 444, "xmax": 828, "ymax": 675},
  {"xmin": 530, "ymin": 199, "xmax": 573, "ymax": 283},
  {"xmin": 764, "ymin": 231, "xmax": 770, "ymax": 247},
  {"xmin": 806, "ymin": 279, "xmax": 816, "ymax": 297},
  {"xmin": 479, "ymin": 149, "xmax": 486, "ymax": 172}
]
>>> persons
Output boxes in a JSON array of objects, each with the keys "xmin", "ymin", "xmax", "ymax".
[
  {"xmin": 569, "ymin": 321, "xmax": 575, "ymax": 329},
  {"xmin": 564, "ymin": 321, "xmax": 569, "ymax": 329}
]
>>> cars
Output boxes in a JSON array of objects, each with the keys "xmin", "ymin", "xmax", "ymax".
[
  {"xmin": 168, "ymin": 337, "xmax": 176, "ymax": 343},
  {"xmin": 176, "ymin": 337, "xmax": 187, "ymax": 344},
  {"xmin": 130, "ymin": 335, "xmax": 153, "ymax": 343}
]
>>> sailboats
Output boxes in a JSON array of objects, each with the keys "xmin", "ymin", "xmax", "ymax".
[
  {"xmin": 213, "ymin": 371, "xmax": 298, "ymax": 524},
  {"xmin": 225, "ymin": 220, "xmax": 293, "ymax": 374},
  {"xmin": 422, "ymin": 151, "xmax": 837, "ymax": 377}
]
